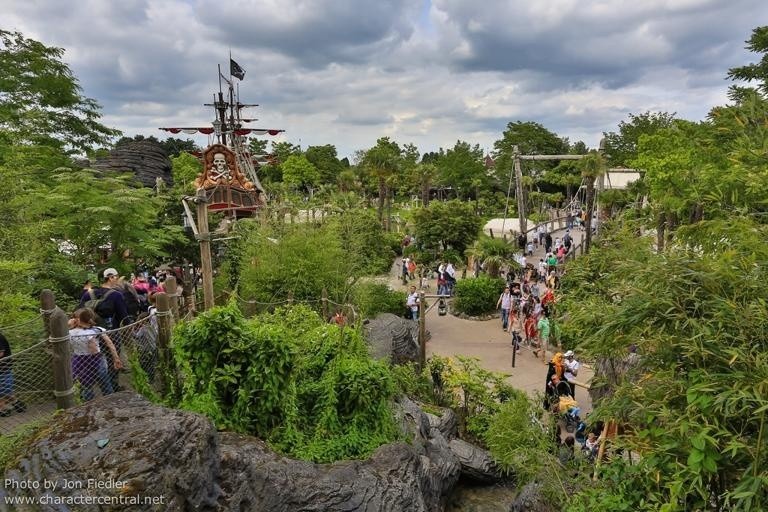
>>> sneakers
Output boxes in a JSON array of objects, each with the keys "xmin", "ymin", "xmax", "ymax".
[{"xmin": 0, "ymin": 401, "xmax": 26, "ymax": 417}]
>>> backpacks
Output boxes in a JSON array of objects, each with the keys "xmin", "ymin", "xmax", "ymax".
[{"xmin": 85, "ymin": 288, "xmax": 116, "ymax": 328}]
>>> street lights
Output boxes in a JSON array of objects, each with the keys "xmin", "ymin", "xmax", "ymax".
[{"xmin": 416, "ymin": 291, "xmax": 452, "ymax": 370}]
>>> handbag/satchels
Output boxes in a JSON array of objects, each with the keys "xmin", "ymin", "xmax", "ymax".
[{"xmin": 404, "ymin": 307, "xmax": 413, "ymax": 319}]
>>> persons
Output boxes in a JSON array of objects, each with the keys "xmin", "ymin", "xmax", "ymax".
[
  {"xmin": 0, "ymin": 332, "xmax": 28, "ymax": 416},
  {"xmin": 395, "ymin": 232, "xmax": 484, "ymax": 322},
  {"xmin": 495, "ymin": 195, "xmax": 646, "ymax": 467},
  {"xmin": 328, "ymin": 305, "xmax": 347, "ymax": 326},
  {"xmin": 64, "ymin": 256, "xmax": 202, "ymax": 403}
]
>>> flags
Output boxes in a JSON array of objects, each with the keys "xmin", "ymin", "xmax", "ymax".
[{"xmin": 230, "ymin": 58, "xmax": 247, "ymax": 81}]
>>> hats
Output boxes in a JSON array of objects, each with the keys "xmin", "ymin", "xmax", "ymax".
[
  {"xmin": 103, "ymin": 268, "xmax": 118, "ymax": 278},
  {"xmin": 563, "ymin": 350, "xmax": 574, "ymax": 357}
]
